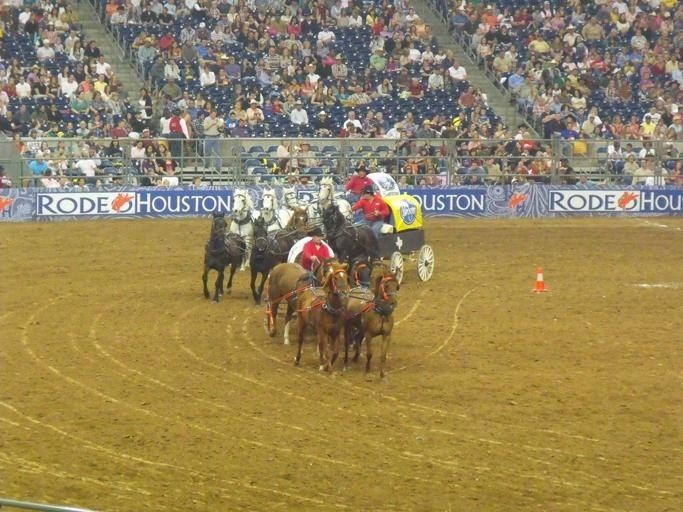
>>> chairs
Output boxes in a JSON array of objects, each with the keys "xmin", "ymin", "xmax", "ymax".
[{"xmin": 0, "ymin": 0, "xmax": 683, "ymax": 192}]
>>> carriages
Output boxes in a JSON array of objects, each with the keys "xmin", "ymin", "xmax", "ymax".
[
  {"xmin": 225, "ymin": 173, "xmax": 399, "ymax": 273},
  {"xmin": 264, "ymin": 236, "xmax": 401, "ymax": 383},
  {"xmin": 200, "ymin": 194, "xmax": 434, "ymax": 307}
]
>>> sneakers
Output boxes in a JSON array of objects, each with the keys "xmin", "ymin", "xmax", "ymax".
[{"xmin": 316, "ymin": 110, "xmax": 327, "ymax": 117}]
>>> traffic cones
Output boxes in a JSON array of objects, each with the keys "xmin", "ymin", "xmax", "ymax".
[{"xmin": 531, "ymin": 266, "xmax": 548, "ymax": 292}]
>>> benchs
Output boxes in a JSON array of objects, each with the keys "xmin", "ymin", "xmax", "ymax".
[{"xmin": 378, "ymin": 222, "xmax": 394, "ymax": 235}]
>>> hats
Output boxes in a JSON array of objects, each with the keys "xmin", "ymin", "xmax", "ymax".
[
  {"xmin": 649, "ymin": 12, "xmax": 658, "ymax": 17},
  {"xmin": 334, "ymin": 55, "xmax": 342, "ymax": 60},
  {"xmin": 294, "ymin": 99, "xmax": 304, "ymax": 106},
  {"xmin": 170, "ymin": 107, "xmax": 184, "ymax": 115},
  {"xmin": 353, "ymin": 165, "xmax": 371, "ymax": 176},
  {"xmin": 361, "ymin": 186, "xmax": 375, "ymax": 194},
  {"xmin": 422, "ymin": 119, "xmax": 433, "ymax": 125},
  {"xmin": 644, "ymin": 115, "xmax": 651, "ymax": 119},
  {"xmin": 305, "ymin": 227, "xmax": 325, "ymax": 238},
  {"xmin": 485, "ymin": 5, "xmax": 493, "ymax": 11},
  {"xmin": 247, "ymin": 99, "xmax": 258, "ymax": 105},
  {"xmin": 516, "ymin": 122, "xmax": 528, "ymax": 129},
  {"xmin": 456, "ymin": 6, "xmax": 466, "ymax": 12}
]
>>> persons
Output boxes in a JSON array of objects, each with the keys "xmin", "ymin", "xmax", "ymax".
[
  {"xmin": 349, "ymin": 185, "xmax": 389, "ymax": 242},
  {"xmin": 302, "ymin": 228, "xmax": 329, "ymax": 270},
  {"xmin": 1, "ymin": 0, "xmax": 683, "ymax": 187},
  {"xmin": 344, "ymin": 167, "xmax": 373, "ymax": 220}
]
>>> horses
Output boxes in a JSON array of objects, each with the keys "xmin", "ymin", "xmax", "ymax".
[
  {"xmin": 201, "ymin": 211, "xmax": 247, "ymax": 303},
  {"xmin": 229, "ymin": 187, "xmax": 261, "ymax": 270},
  {"xmin": 246, "ymin": 174, "xmax": 401, "ymax": 383}
]
